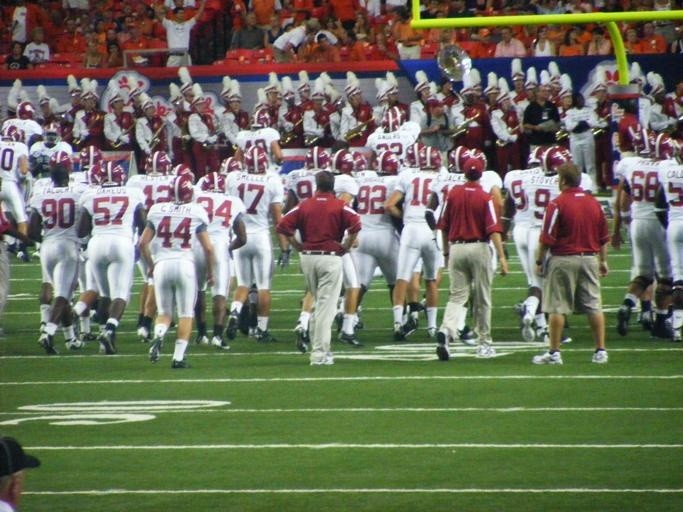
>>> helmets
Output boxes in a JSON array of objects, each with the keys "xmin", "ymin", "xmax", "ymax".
[
  {"xmin": 304, "ymin": 146, "xmax": 367, "ymax": 174},
  {"xmin": 145, "ymin": 150, "xmax": 171, "ymax": 176},
  {"xmin": 527, "ymin": 146, "xmax": 550, "ymax": 165},
  {"xmin": 1, "ymin": 125, "xmax": 23, "ymax": 142},
  {"xmin": 632, "ymin": 128, "xmax": 683, "ymax": 160},
  {"xmin": 376, "ymin": 150, "xmax": 399, "ymax": 175},
  {"xmin": 50, "ymin": 145, "xmax": 125, "ymax": 187},
  {"xmin": 406, "ymin": 142, "xmax": 486, "ymax": 173},
  {"xmin": 167, "ymin": 145, "xmax": 269, "ymax": 203},
  {"xmin": 40, "ymin": 122, "xmax": 62, "ymax": 148},
  {"xmin": 542, "ymin": 145, "xmax": 574, "ymax": 176},
  {"xmin": 251, "ymin": 105, "xmax": 274, "ymax": 128}
]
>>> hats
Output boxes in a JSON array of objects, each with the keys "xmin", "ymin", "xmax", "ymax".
[
  {"xmin": 7, "ymin": 74, "xmax": 154, "ymax": 117},
  {"xmin": 169, "ymin": 66, "xmax": 241, "ymax": 106},
  {"xmin": 0, "ymin": 436, "xmax": 40, "ymax": 477},
  {"xmin": 255, "ymin": 70, "xmax": 346, "ymax": 111},
  {"xmin": 459, "ymin": 58, "xmax": 573, "ymax": 104},
  {"xmin": 591, "ymin": 62, "xmax": 665, "ymax": 97},
  {"xmin": 344, "ymin": 71, "xmax": 431, "ymax": 103}
]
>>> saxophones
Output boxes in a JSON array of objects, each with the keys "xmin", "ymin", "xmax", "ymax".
[
  {"xmin": 450, "ymin": 89, "xmax": 479, "ymax": 137},
  {"xmin": 112, "ymin": 113, "xmax": 137, "ymax": 147},
  {"xmin": 591, "ymin": 100, "xmax": 611, "ymax": 135},
  {"xmin": 73, "ymin": 106, "xmax": 101, "ymax": 146},
  {"xmin": 344, "ymin": 95, "xmax": 376, "ymax": 139},
  {"xmin": 279, "ymin": 105, "xmax": 303, "ymax": 146},
  {"xmin": 496, "ymin": 101, "xmax": 524, "ymax": 147},
  {"xmin": 146, "ymin": 116, "xmax": 167, "ymax": 151},
  {"xmin": 304, "ymin": 106, "xmax": 330, "ymax": 146}
]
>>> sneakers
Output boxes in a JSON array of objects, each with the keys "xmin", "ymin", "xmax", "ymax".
[
  {"xmin": 137, "ymin": 326, "xmax": 231, "ymax": 368},
  {"xmin": 37, "ymin": 309, "xmax": 118, "ymax": 355},
  {"xmin": 226, "ymin": 303, "xmax": 277, "ymax": 342},
  {"xmin": 394, "ymin": 298, "xmax": 437, "ymax": 340},
  {"xmin": 6, "ymin": 242, "xmax": 40, "ymax": 260},
  {"xmin": 617, "ymin": 305, "xmax": 683, "ymax": 342},
  {"xmin": 293, "ymin": 310, "xmax": 364, "ymax": 365},
  {"xmin": 477, "ymin": 345, "xmax": 496, "ymax": 357},
  {"xmin": 515, "ymin": 304, "xmax": 608, "ymax": 364},
  {"xmin": 435, "ymin": 329, "xmax": 449, "ymax": 360}
]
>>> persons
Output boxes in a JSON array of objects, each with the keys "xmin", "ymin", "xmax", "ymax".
[
  {"xmin": 0, "ymin": 437, "xmax": 41, "ymax": 512},
  {"xmin": 0, "ymin": 0, "xmax": 683, "ymax": 368}
]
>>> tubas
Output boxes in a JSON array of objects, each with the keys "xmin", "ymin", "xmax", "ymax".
[{"xmin": 437, "ymin": 44, "xmax": 473, "ymax": 105}]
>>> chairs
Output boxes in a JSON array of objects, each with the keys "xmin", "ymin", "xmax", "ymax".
[{"xmin": 0, "ymin": 0, "xmax": 392, "ymax": 62}]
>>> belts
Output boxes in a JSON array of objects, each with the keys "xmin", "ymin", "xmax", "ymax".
[
  {"xmin": 167, "ymin": 53, "xmax": 185, "ymax": 60},
  {"xmin": 452, "ymin": 239, "xmax": 489, "ymax": 244},
  {"xmin": 574, "ymin": 253, "xmax": 595, "ymax": 256},
  {"xmin": 302, "ymin": 252, "xmax": 341, "ymax": 256}
]
